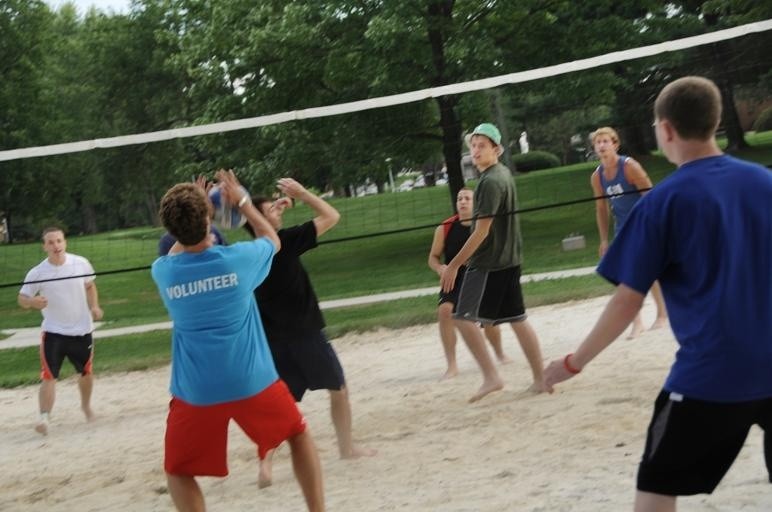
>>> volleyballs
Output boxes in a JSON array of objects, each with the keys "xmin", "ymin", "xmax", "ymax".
[{"xmin": 207, "ymin": 184, "xmax": 252, "ymax": 231}]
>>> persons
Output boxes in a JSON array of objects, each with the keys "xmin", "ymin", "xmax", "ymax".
[
  {"xmin": 17, "ymin": 224, "xmax": 105, "ymax": 436},
  {"xmin": 427, "ymin": 186, "xmax": 515, "ymax": 384},
  {"xmin": 586, "ymin": 126, "xmax": 672, "ymax": 344},
  {"xmin": 538, "ymin": 74, "xmax": 770, "ymax": 511},
  {"xmin": 438, "ymin": 121, "xmax": 555, "ymax": 402}
]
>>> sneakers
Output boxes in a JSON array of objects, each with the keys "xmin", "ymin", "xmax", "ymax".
[{"xmin": 34, "ymin": 410, "xmax": 50, "ymax": 436}]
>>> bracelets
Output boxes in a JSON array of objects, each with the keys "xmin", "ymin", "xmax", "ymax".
[{"xmin": 564, "ymin": 353, "xmax": 582, "ymax": 374}]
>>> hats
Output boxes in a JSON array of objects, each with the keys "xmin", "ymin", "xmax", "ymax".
[{"xmin": 464, "ymin": 123, "xmax": 502, "ymax": 149}]
